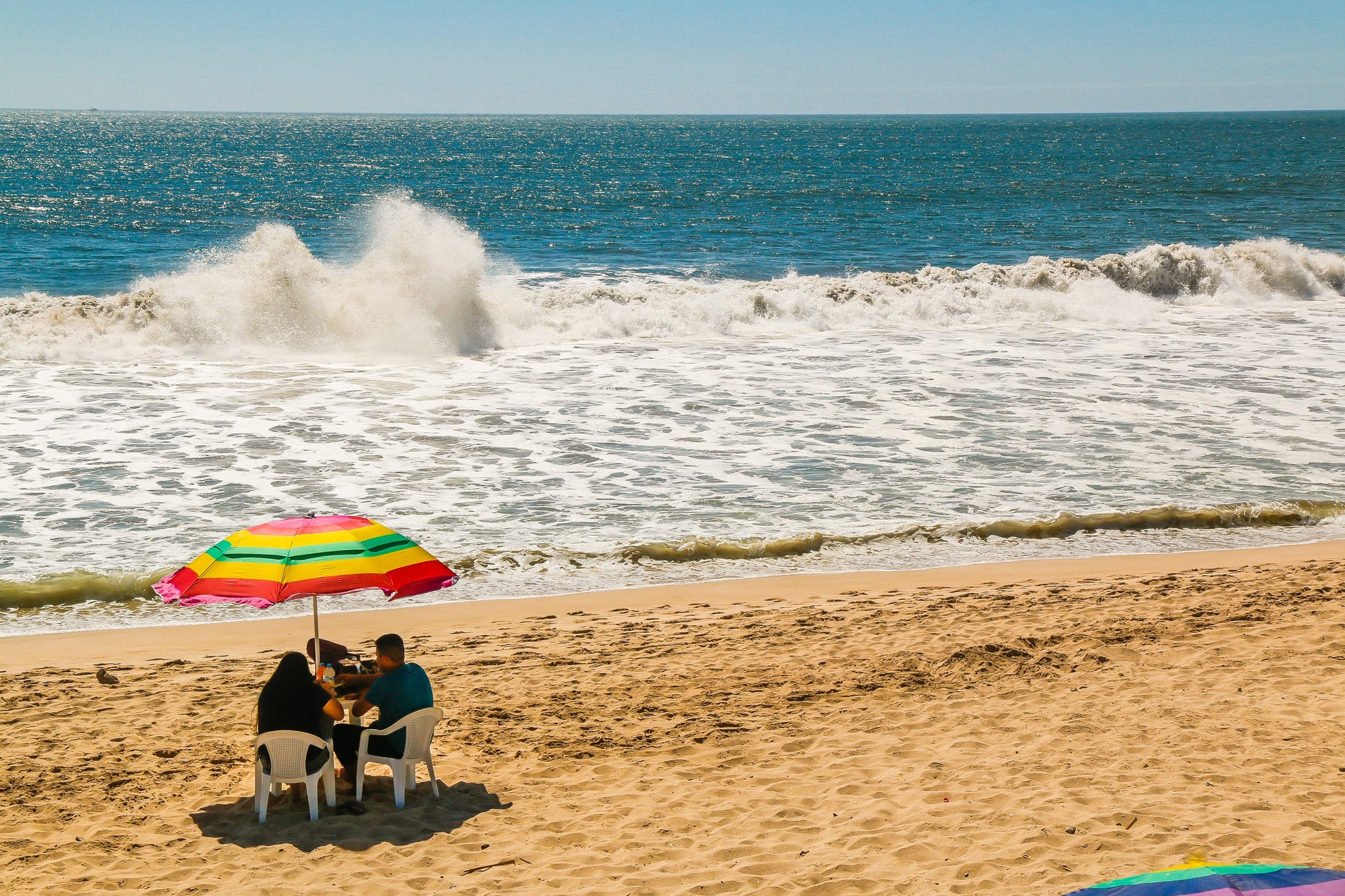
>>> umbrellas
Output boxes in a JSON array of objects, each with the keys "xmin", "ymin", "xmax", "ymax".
[{"xmin": 150, "ymin": 512, "xmax": 460, "ymax": 684}]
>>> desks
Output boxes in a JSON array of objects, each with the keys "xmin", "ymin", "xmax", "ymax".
[{"xmin": 334, "ymin": 696, "xmax": 365, "ymax": 727}]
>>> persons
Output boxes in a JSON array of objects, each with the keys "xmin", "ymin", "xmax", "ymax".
[
  {"xmin": 252, "ymin": 651, "xmax": 344, "ymax": 808},
  {"xmin": 332, "ymin": 633, "xmax": 435, "ymax": 784}
]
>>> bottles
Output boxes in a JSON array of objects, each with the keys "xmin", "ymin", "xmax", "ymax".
[
  {"xmin": 323, "ymin": 664, "xmax": 335, "ymax": 696},
  {"xmin": 317, "ymin": 663, "xmax": 326, "ymax": 680}
]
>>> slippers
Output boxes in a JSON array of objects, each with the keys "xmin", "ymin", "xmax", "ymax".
[
  {"xmin": 344, "ymin": 801, "xmax": 367, "ymax": 815},
  {"xmin": 336, "ymin": 804, "xmax": 355, "ymax": 816}
]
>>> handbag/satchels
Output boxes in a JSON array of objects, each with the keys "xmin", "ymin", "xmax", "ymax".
[{"xmin": 359, "ymin": 659, "xmax": 380, "ymax": 675}]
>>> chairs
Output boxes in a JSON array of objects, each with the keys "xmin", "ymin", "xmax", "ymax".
[
  {"xmin": 255, "ymin": 729, "xmax": 336, "ymax": 822},
  {"xmin": 356, "ymin": 707, "xmax": 444, "ymax": 807}
]
